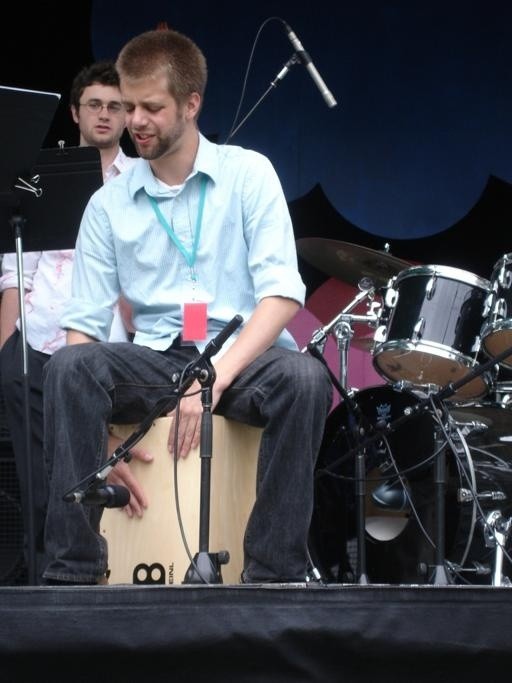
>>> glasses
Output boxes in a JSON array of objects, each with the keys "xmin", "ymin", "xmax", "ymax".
[{"xmin": 79, "ymin": 101, "xmax": 127, "ymax": 115}]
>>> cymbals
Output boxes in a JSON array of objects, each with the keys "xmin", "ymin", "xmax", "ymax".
[{"xmin": 291, "ymin": 239, "xmax": 414, "ymax": 283}]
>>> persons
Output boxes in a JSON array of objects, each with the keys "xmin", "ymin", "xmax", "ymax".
[
  {"xmin": 0, "ymin": 57, "xmax": 143, "ymax": 589},
  {"xmin": 32, "ymin": 27, "xmax": 333, "ymax": 585}
]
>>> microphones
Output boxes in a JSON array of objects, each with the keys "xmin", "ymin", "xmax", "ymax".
[
  {"xmin": 63, "ymin": 485, "xmax": 130, "ymax": 508},
  {"xmin": 284, "ymin": 24, "xmax": 337, "ymax": 108}
]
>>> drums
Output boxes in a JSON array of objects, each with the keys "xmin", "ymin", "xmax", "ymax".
[
  {"xmin": 101, "ymin": 412, "xmax": 263, "ymax": 588},
  {"xmin": 370, "ymin": 263, "xmax": 498, "ymax": 398},
  {"xmin": 306, "ymin": 384, "xmax": 509, "ymax": 585},
  {"xmin": 480, "ymin": 255, "xmax": 512, "ymax": 368}
]
